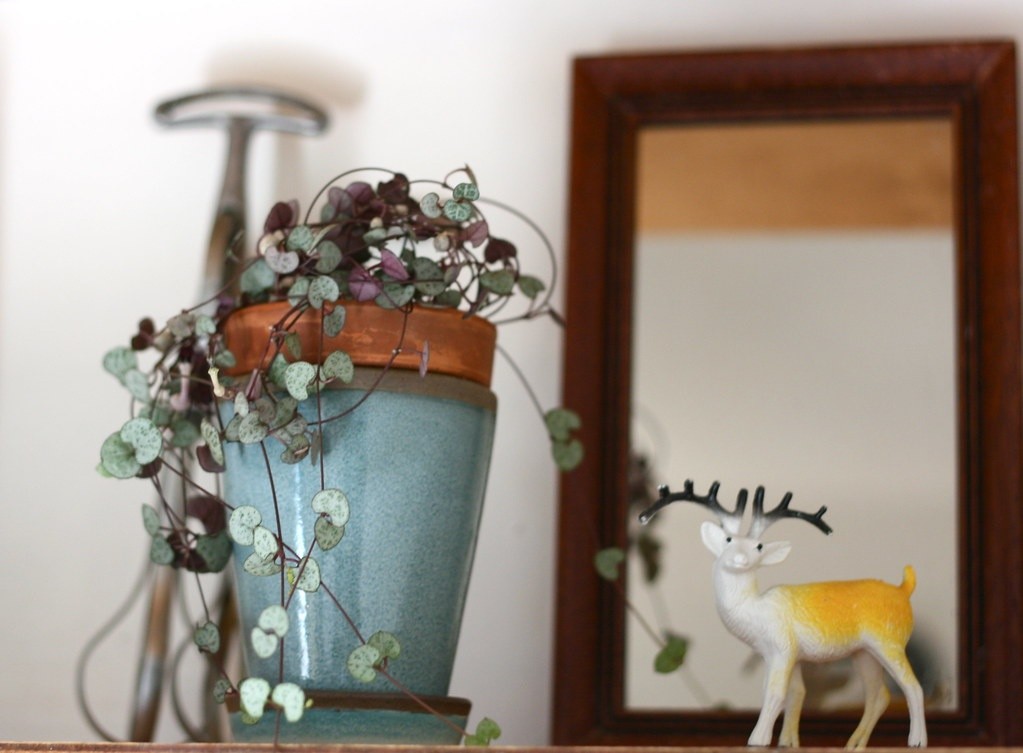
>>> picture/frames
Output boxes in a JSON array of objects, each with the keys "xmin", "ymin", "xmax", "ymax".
[{"xmin": 541, "ymin": 34, "xmax": 1023, "ymax": 747}]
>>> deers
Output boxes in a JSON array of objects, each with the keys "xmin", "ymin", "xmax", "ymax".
[{"xmin": 638, "ymin": 478, "xmax": 929, "ymax": 752}]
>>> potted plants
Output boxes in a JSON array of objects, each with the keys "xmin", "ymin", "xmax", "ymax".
[{"xmin": 93, "ymin": 158, "xmax": 695, "ymax": 743}]
